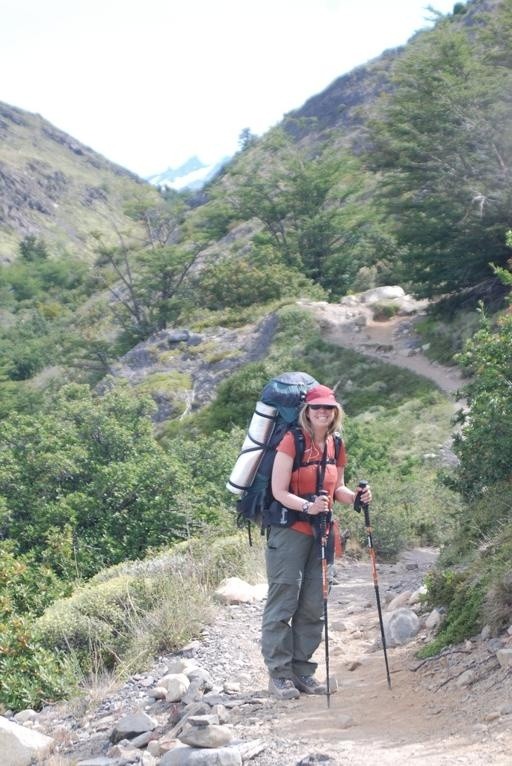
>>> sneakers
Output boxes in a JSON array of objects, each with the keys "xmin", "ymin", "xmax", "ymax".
[
  {"xmin": 266, "ymin": 675, "xmax": 300, "ymax": 701},
  {"xmin": 292, "ymin": 672, "xmax": 327, "ymax": 695}
]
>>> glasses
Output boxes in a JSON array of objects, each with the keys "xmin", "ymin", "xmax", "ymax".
[{"xmin": 309, "ymin": 403, "xmax": 336, "ymax": 410}]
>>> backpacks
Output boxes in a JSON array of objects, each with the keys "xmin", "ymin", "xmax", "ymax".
[{"xmin": 235, "ymin": 371, "xmax": 345, "ymax": 526}]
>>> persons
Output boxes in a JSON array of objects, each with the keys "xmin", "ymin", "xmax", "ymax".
[{"xmin": 259, "ymin": 384, "xmax": 373, "ymax": 700}]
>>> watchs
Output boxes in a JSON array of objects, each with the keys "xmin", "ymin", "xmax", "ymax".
[{"xmin": 302, "ymin": 502, "xmax": 310, "ymax": 514}]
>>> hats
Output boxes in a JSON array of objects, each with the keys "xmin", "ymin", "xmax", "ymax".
[{"xmin": 304, "ymin": 384, "xmax": 336, "ymax": 406}]
